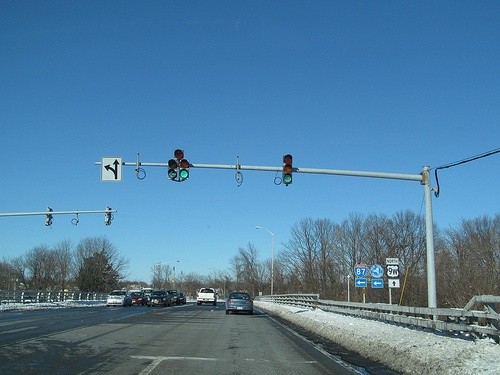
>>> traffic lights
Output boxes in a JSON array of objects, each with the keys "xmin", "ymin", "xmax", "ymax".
[
  {"xmin": 167, "ymin": 149, "xmax": 189, "ymax": 182},
  {"xmin": 45, "ymin": 208, "xmax": 53, "ymax": 226},
  {"xmin": 282, "ymin": 154, "xmax": 293, "ymax": 185}
]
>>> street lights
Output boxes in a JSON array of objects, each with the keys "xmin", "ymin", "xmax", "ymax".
[
  {"xmin": 255, "ymin": 226, "xmax": 275, "ymax": 300},
  {"xmin": 153, "ymin": 260, "xmax": 180, "ymax": 289}
]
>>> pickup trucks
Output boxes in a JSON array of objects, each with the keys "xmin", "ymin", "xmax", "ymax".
[{"xmin": 196, "ymin": 288, "xmax": 219, "ymax": 306}]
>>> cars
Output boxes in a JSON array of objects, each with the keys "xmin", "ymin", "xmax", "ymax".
[
  {"xmin": 224, "ymin": 291, "xmax": 254, "ymax": 315},
  {"xmin": 106, "ymin": 290, "xmax": 132, "ymax": 307},
  {"xmin": 130, "ymin": 287, "xmax": 186, "ymax": 308}
]
